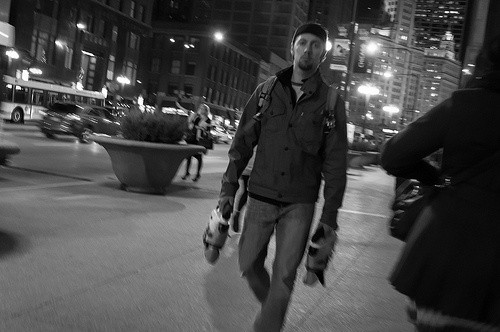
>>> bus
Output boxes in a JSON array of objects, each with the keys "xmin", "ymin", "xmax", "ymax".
[{"xmin": 0, "ymin": 74, "xmax": 106, "ymax": 123}]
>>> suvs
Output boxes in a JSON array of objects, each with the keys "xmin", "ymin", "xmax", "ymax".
[{"xmin": 40, "ymin": 102, "xmax": 121, "ymax": 144}]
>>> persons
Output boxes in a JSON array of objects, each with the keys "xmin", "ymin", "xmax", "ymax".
[
  {"xmin": 52, "ymin": 95, "xmax": 59, "ymax": 100},
  {"xmin": 15, "ymin": 90, "xmax": 25, "ymax": 102},
  {"xmin": 232, "ymin": 144, "xmax": 257, "ymax": 233},
  {"xmin": 33, "ymin": 92, "xmax": 43, "ymax": 104},
  {"xmin": 203, "ymin": 22, "xmax": 348, "ymax": 332},
  {"xmin": 182, "ymin": 104, "xmax": 214, "ymax": 182},
  {"xmin": 381, "ymin": 36, "xmax": 500, "ymax": 332}
]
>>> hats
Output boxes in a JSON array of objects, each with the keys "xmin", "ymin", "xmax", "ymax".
[{"xmin": 293, "ymin": 23, "xmax": 327, "ymax": 43}]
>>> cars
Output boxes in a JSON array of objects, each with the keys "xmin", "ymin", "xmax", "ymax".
[
  {"xmin": 346, "ymin": 123, "xmax": 400, "ymax": 154},
  {"xmin": 111, "ymin": 97, "xmax": 239, "ymax": 145}
]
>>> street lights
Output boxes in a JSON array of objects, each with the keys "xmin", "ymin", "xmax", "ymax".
[
  {"xmin": 365, "ymin": 41, "xmax": 420, "ymax": 128},
  {"xmin": 178, "ymin": 29, "xmax": 224, "ymax": 96}
]
